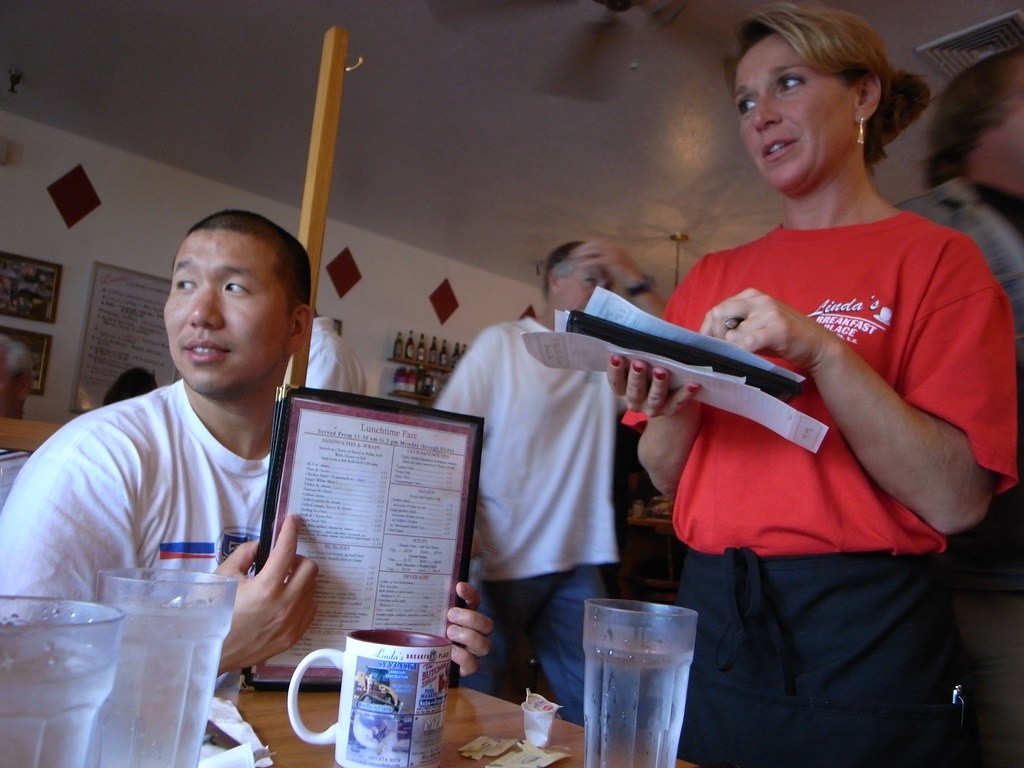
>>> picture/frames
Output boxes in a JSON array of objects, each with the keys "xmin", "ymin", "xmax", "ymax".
[
  {"xmin": 0, "ymin": 250, "xmax": 63, "ymax": 324},
  {"xmin": 0, "ymin": 326, "xmax": 51, "ymax": 396}
]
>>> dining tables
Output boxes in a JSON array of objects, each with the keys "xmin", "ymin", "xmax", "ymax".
[{"xmin": 214, "ymin": 685, "xmax": 701, "ymax": 768}]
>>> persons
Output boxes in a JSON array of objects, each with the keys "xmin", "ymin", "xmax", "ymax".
[
  {"xmin": 104, "ymin": 367, "xmax": 157, "ymax": 406},
  {"xmin": 607, "ymin": 7, "xmax": 1018, "ymax": 768},
  {"xmin": 433, "ymin": 242, "xmax": 665, "ymax": 727},
  {"xmin": 0, "ymin": 335, "xmax": 34, "ymax": 514},
  {"xmin": 283, "ymin": 306, "xmax": 366, "ymax": 395},
  {"xmin": 892, "ymin": 45, "xmax": 1024, "ymax": 768},
  {"xmin": 0, "ymin": 208, "xmax": 496, "ymax": 687}
]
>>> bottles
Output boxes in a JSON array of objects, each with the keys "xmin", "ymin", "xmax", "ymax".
[
  {"xmin": 439, "ymin": 340, "xmax": 446, "ymax": 366},
  {"xmin": 429, "ymin": 336, "xmax": 437, "ymax": 364},
  {"xmin": 405, "ymin": 331, "xmax": 414, "ymax": 359},
  {"xmin": 451, "ymin": 343, "xmax": 460, "ymax": 368},
  {"xmin": 418, "ymin": 334, "xmax": 425, "ymax": 361},
  {"xmin": 460, "ymin": 345, "xmax": 466, "ymax": 356},
  {"xmin": 394, "ymin": 332, "xmax": 403, "ymax": 358},
  {"xmin": 393, "ymin": 365, "xmax": 443, "ymax": 397}
]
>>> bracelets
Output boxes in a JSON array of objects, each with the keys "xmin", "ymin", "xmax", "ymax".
[{"xmin": 623, "ymin": 275, "xmax": 652, "ymax": 294}]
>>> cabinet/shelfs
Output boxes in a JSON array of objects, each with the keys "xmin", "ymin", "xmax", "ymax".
[{"xmin": 388, "ymin": 356, "xmax": 455, "ymax": 401}]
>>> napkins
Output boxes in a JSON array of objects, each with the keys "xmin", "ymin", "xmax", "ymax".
[{"xmin": 198, "ymin": 696, "xmax": 275, "ymax": 768}]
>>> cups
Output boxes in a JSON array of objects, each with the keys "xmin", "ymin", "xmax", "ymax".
[
  {"xmin": 85, "ymin": 568, "xmax": 239, "ymax": 768},
  {"xmin": 0, "ymin": 595, "xmax": 127, "ymax": 767},
  {"xmin": 582, "ymin": 598, "xmax": 698, "ymax": 767},
  {"xmin": 287, "ymin": 628, "xmax": 453, "ymax": 768},
  {"xmin": 521, "ymin": 702, "xmax": 559, "ymax": 748}
]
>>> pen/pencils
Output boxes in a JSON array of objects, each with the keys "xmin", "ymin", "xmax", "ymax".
[{"xmin": 722, "ymin": 316, "xmax": 745, "ymax": 328}]
